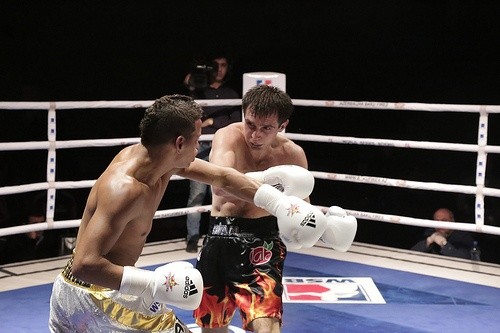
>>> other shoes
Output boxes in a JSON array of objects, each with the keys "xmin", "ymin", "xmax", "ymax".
[{"xmin": 186, "ymin": 241, "xmax": 198, "ymax": 253}]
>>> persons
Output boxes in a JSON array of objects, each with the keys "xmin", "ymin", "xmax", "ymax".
[
  {"xmin": 191, "ymin": 85, "xmax": 358, "ymax": 333},
  {"xmin": 8, "ymin": 208, "xmax": 61, "ymax": 263},
  {"xmin": 48, "ymin": 93, "xmax": 327, "ymax": 333},
  {"xmin": 183, "ymin": 52, "xmax": 243, "ymax": 254},
  {"xmin": 411, "ymin": 209, "xmax": 493, "ymax": 262}
]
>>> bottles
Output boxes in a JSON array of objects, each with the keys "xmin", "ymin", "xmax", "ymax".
[{"xmin": 470, "ymin": 241, "xmax": 481, "ymax": 272}]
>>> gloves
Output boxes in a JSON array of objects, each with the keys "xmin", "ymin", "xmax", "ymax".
[
  {"xmin": 244, "ymin": 164, "xmax": 315, "ymax": 200},
  {"xmin": 253, "ymin": 184, "xmax": 327, "ymax": 250},
  {"xmin": 313, "ymin": 206, "xmax": 358, "ymax": 252},
  {"xmin": 117, "ymin": 261, "xmax": 204, "ymax": 311}
]
